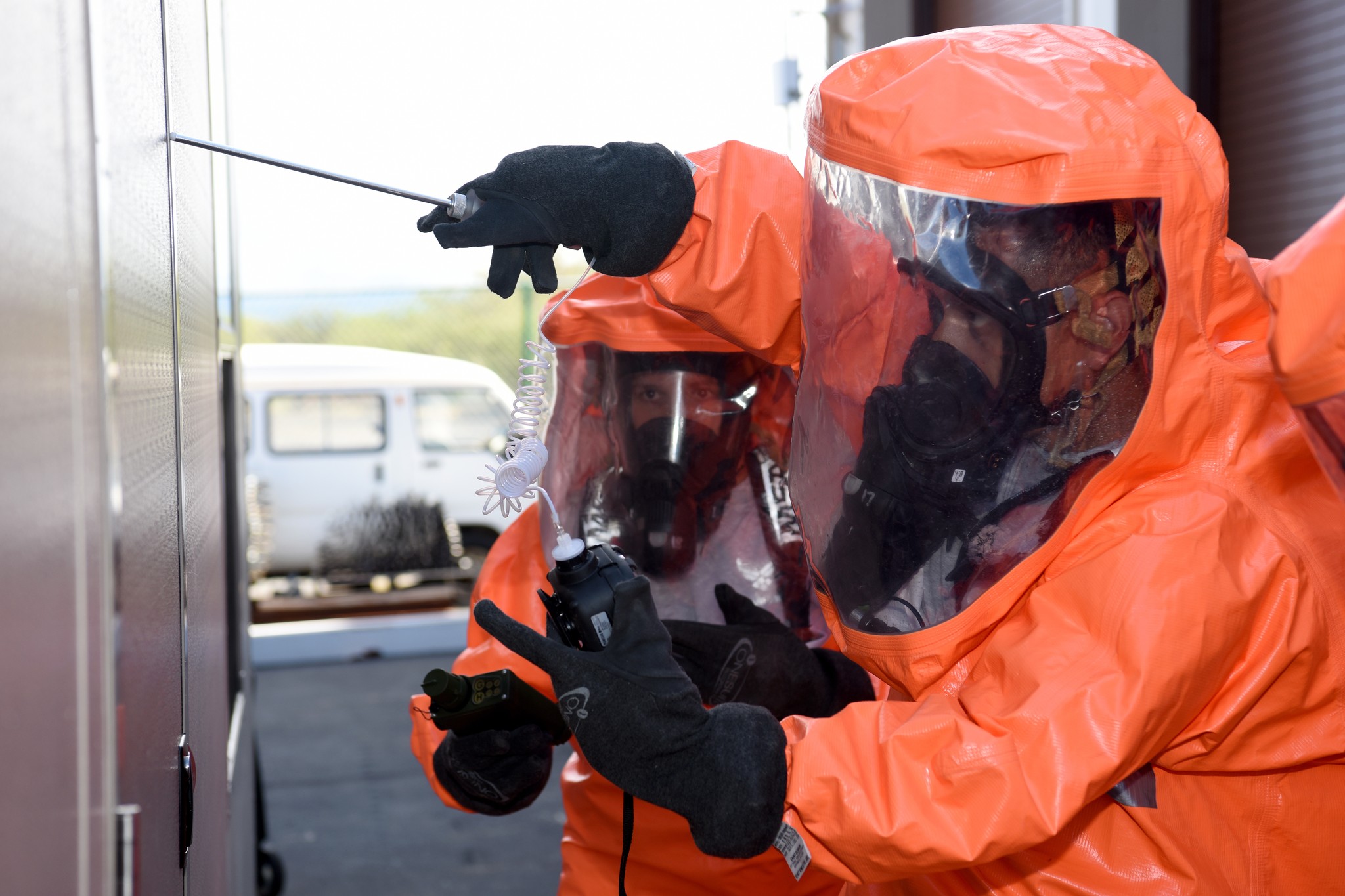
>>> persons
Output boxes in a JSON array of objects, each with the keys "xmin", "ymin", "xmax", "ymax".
[
  {"xmin": 409, "ymin": 267, "xmax": 874, "ymax": 896},
  {"xmin": 416, "ymin": 22, "xmax": 1345, "ymax": 896}
]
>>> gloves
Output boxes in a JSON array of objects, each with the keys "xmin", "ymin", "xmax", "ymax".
[
  {"xmin": 418, "ymin": 139, "xmax": 696, "ymax": 301},
  {"xmin": 421, "ymin": 668, "xmax": 570, "ymax": 817},
  {"xmin": 472, "ymin": 576, "xmax": 790, "ymax": 857},
  {"xmin": 658, "ymin": 582, "xmax": 878, "ymax": 720}
]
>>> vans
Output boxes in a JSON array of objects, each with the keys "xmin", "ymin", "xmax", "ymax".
[{"xmin": 242, "ymin": 341, "xmax": 540, "ymax": 603}]
